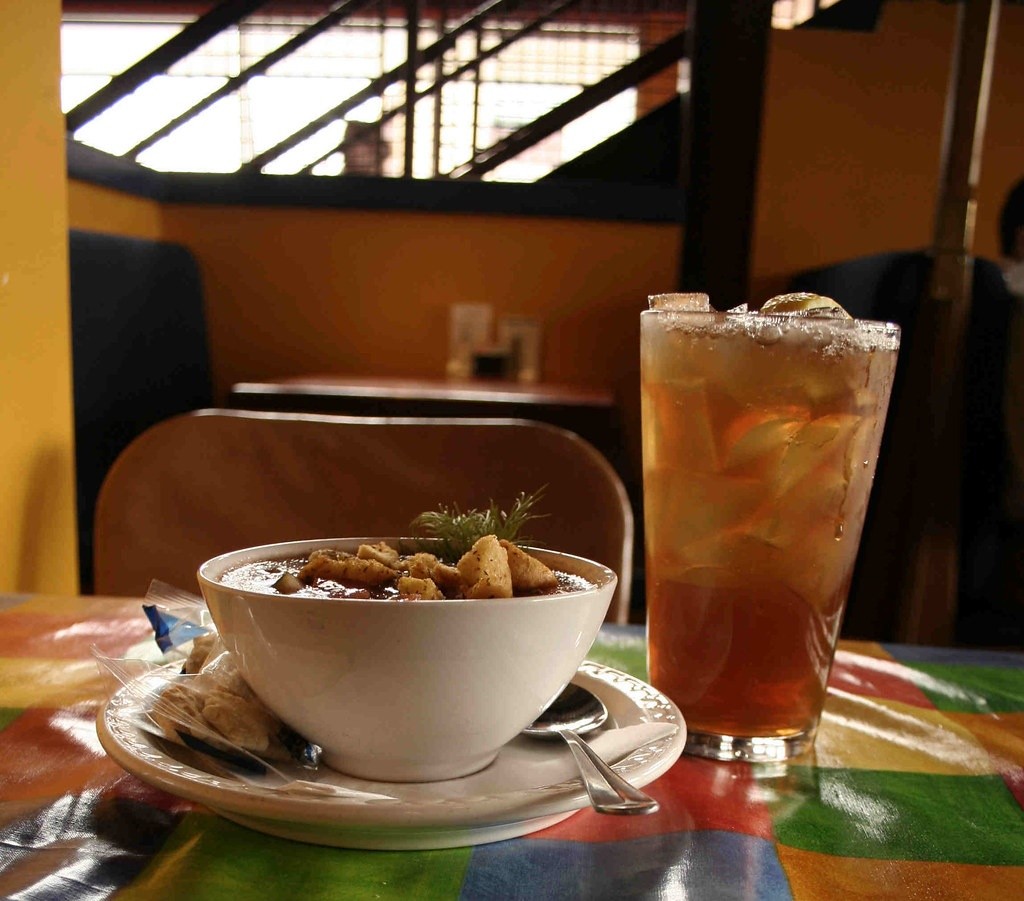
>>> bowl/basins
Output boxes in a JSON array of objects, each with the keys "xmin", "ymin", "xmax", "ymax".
[
  {"xmin": 196, "ymin": 538, "xmax": 618, "ymax": 782},
  {"xmin": 472, "ymin": 354, "xmax": 508, "ymax": 377}
]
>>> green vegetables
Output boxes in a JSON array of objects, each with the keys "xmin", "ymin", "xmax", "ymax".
[{"xmin": 409, "ymin": 484, "xmax": 552, "ymax": 559}]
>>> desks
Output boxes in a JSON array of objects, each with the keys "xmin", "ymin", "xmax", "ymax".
[{"xmin": 227, "ymin": 372, "xmax": 619, "ymax": 411}]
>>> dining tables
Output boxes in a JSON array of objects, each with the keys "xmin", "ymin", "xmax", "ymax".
[{"xmin": 1, "ymin": 594, "xmax": 1024, "ymax": 901}]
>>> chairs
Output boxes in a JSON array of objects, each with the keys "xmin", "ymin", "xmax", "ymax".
[{"xmin": 91, "ymin": 409, "xmax": 631, "ymax": 627}]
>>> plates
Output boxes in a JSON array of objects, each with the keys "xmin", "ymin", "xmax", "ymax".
[{"xmin": 95, "ymin": 660, "xmax": 686, "ymax": 849}]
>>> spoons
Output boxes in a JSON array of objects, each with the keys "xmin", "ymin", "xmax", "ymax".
[{"xmin": 522, "ymin": 682, "xmax": 661, "ymax": 816}]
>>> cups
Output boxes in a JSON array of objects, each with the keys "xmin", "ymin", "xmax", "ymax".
[
  {"xmin": 498, "ymin": 321, "xmax": 542, "ymax": 384},
  {"xmin": 642, "ymin": 307, "xmax": 902, "ymax": 763},
  {"xmin": 447, "ymin": 302, "xmax": 491, "ymax": 380}
]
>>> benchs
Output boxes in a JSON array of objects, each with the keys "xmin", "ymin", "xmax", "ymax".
[
  {"xmin": 632, "ymin": 250, "xmax": 1024, "ymax": 653},
  {"xmin": 69, "ymin": 227, "xmax": 212, "ymax": 595}
]
